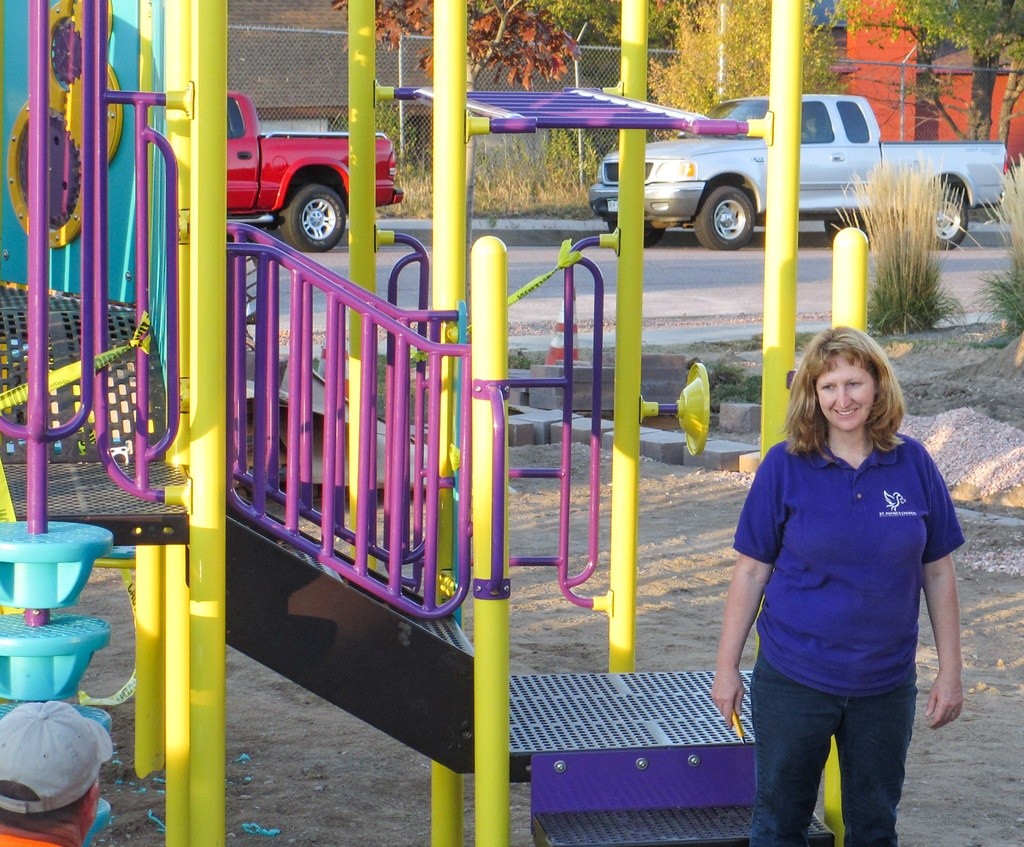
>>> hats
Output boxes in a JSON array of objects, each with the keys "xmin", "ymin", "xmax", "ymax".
[{"xmin": 0, "ymin": 699, "xmax": 113, "ymax": 813}]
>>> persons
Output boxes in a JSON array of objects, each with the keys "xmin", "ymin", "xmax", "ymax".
[
  {"xmin": 710, "ymin": 326, "xmax": 966, "ymax": 846},
  {"xmin": 0, "ymin": 699, "xmax": 115, "ymax": 847}
]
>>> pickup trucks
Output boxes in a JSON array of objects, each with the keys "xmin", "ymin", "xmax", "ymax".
[
  {"xmin": 227, "ymin": 91, "xmax": 405, "ymax": 253},
  {"xmin": 589, "ymin": 94, "xmax": 1007, "ymax": 251}
]
